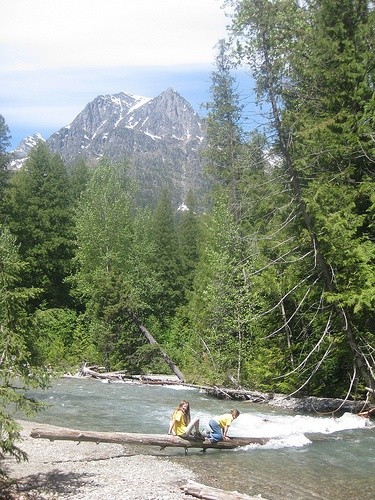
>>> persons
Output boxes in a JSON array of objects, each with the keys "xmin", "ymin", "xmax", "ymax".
[
  {"xmin": 169, "ymin": 400, "xmax": 205, "ymax": 441},
  {"xmin": 200, "ymin": 409, "xmax": 240, "ymax": 445}
]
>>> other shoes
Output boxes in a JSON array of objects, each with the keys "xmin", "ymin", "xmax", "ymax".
[
  {"xmin": 194, "ymin": 432, "xmax": 205, "ymax": 440},
  {"xmin": 200, "ymin": 428, "xmax": 206, "ymax": 436}
]
